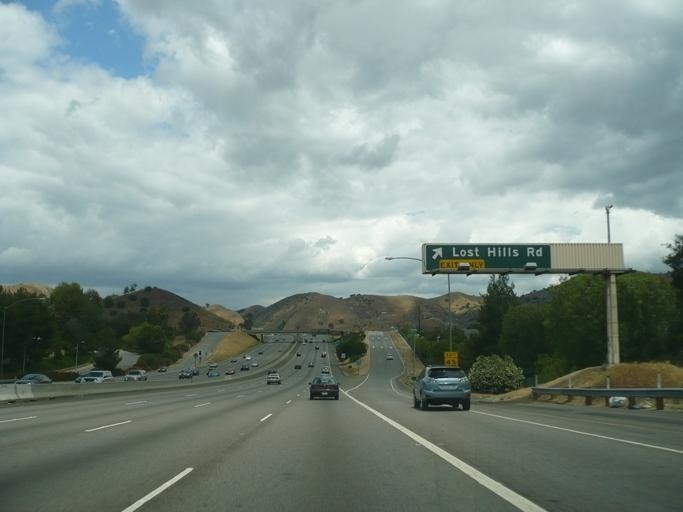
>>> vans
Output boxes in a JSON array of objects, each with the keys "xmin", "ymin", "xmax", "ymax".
[{"xmin": 409, "ymin": 364, "xmax": 472, "ymax": 413}]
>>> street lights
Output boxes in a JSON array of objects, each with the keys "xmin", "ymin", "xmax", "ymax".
[
  {"xmin": 601, "ymin": 202, "xmax": 613, "ymax": 243},
  {"xmin": 377, "ymin": 256, "xmax": 452, "ymax": 351},
  {"xmin": 76, "ymin": 341, "xmax": 84, "ymax": 375},
  {"xmin": 411, "ymin": 333, "xmax": 419, "ymax": 372},
  {"xmin": 0, "ymin": 296, "xmax": 47, "ymax": 378}
]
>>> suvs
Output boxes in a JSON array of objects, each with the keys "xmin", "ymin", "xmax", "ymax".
[{"xmin": 75, "ymin": 369, "xmax": 113, "ymax": 384}]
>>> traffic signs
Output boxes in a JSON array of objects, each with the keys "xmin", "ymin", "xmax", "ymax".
[{"xmin": 422, "ymin": 241, "xmax": 554, "ymax": 274}]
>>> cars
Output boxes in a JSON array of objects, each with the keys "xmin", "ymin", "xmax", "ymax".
[
  {"xmin": 241, "ymin": 363, "xmax": 250, "ymax": 371},
  {"xmin": 385, "ymin": 353, "xmax": 393, "ymax": 360},
  {"xmin": 16, "ymin": 374, "xmax": 54, "ymax": 384},
  {"xmin": 241, "ymin": 352, "xmax": 252, "ymax": 360},
  {"xmin": 296, "ymin": 352, "xmax": 302, "ymax": 357},
  {"xmin": 226, "ymin": 369, "xmax": 235, "ymax": 375},
  {"xmin": 178, "ymin": 368, "xmax": 194, "ymax": 380},
  {"xmin": 309, "ymin": 374, "xmax": 340, "ymax": 401},
  {"xmin": 315, "ymin": 345, "xmax": 320, "ymax": 350},
  {"xmin": 267, "ymin": 373, "xmax": 282, "ymax": 385},
  {"xmin": 205, "ymin": 364, "xmax": 220, "ymax": 378},
  {"xmin": 159, "ymin": 367, "xmax": 167, "ymax": 372},
  {"xmin": 251, "ymin": 361, "xmax": 258, "ymax": 367},
  {"xmin": 308, "ymin": 361, "xmax": 315, "ymax": 368},
  {"xmin": 321, "ymin": 366, "xmax": 331, "ymax": 373},
  {"xmin": 321, "ymin": 352, "xmax": 327, "ymax": 358},
  {"xmin": 294, "ymin": 363, "xmax": 302, "ymax": 369},
  {"xmin": 124, "ymin": 369, "xmax": 147, "ymax": 382}
]
